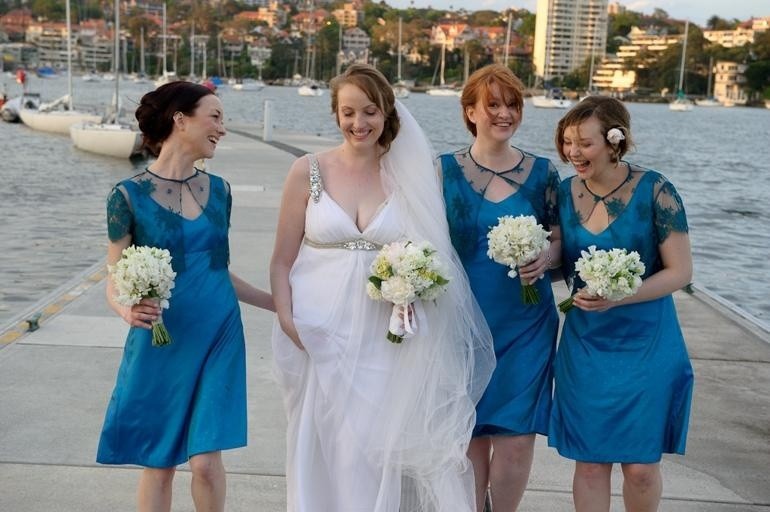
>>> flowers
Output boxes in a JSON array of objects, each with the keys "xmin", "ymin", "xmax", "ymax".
[
  {"xmin": 107, "ymin": 240, "xmax": 178, "ymax": 347},
  {"xmin": 484, "ymin": 212, "xmax": 648, "ymax": 313},
  {"xmin": 369, "ymin": 233, "xmax": 453, "ymax": 344},
  {"xmin": 606, "ymin": 126, "xmax": 624, "ymax": 144}
]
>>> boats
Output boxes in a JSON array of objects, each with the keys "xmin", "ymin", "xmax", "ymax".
[{"xmin": 532, "ymin": 87, "xmax": 572, "ymax": 108}]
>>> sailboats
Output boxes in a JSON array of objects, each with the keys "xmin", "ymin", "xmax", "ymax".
[
  {"xmin": 17, "ymin": 0, "xmax": 104, "ymax": 134},
  {"xmin": 389, "ymin": 18, "xmax": 412, "ymax": 98},
  {"xmin": 68, "ymin": 0, "xmax": 151, "ymax": 159},
  {"xmin": 693, "ymin": 54, "xmax": 724, "ymax": 107},
  {"xmin": 425, "ymin": 43, "xmax": 459, "ymax": 97},
  {"xmin": 82, "ymin": 0, "xmax": 328, "ymax": 98},
  {"xmin": 666, "ymin": 18, "xmax": 693, "ymax": 112}
]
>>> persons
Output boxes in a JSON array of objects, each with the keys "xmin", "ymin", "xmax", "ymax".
[
  {"xmin": 95, "ymin": 78, "xmax": 292, "ymax": 512},
  {"xmin": 432, "ymin": 59, "xmax": 569, "ymax": 512},
  {"xmin": 266, "ymin": 62, "xmax": 502, "ymax": 512},
  {"xmin": 546, "ymin": 92, "xmax": 697, "ymax": 512}
]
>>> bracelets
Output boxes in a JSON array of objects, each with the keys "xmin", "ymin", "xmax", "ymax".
[{"xmin": 547, "ymin": 252, "xmax": 553, "ymax": 270}]
